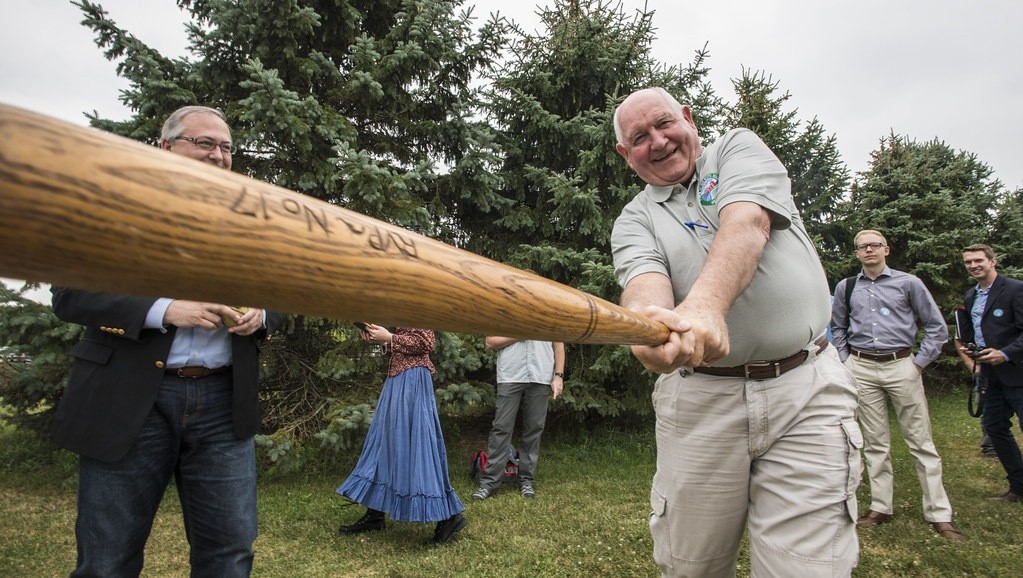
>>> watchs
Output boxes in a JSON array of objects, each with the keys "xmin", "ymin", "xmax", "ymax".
[{"xmin": 555, "ymin": 373, "xmax": 564, "ymax": 377}]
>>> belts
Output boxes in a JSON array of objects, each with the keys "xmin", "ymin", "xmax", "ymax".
[
  {"xmin": 849, "ymin": 347, "xmax": 914, "ymax": 361},
  {"xmin": 164, "ymin": 365, "xmax": 233, "ymax": 378},
  {"xmin": 693, "ymin": 333, "xmax": 829, "ymax": 381}
]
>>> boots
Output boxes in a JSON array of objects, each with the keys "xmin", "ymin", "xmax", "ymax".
[
  {"xmin": 339, "ymin": 508, "xmax": 387, "ymax": 531},
  {"xmin": 434, "ymin": 513, "xmax": 466, "ymax": 545}
]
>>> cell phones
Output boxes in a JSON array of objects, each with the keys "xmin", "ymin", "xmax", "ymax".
[{"xmin": 353, "ymin": 321, "xmax": 374, "ymax": 336}]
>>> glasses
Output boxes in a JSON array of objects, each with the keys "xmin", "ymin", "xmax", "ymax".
[
  {"xmin": 175, "ymin": 135, "xmax": 238, "ymax": 155},
  {"xmin": 857, "ymin": 243, "xmax": 886, "ymax": 250}
]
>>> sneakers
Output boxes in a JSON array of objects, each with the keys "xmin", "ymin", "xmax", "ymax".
[
  {"xmin": 472, "ymin": 487, "xmax": 492, "ymax": 499},
  {"xmin": 521, "ymin": 485, "xmax": 535, "ymax": 498}
]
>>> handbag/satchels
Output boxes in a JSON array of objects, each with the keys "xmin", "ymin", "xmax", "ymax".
[{"xmin": 471, "ymin": 450, "xmax": 521, "ymax": 477}]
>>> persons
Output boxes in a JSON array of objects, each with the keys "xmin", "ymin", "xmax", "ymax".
[
  {"xmin": 832, "ymin": 230, "xmax": 965, "ymax": 545},
  {"xmin": 957, "ymin": 243, "xmax": 1023, "ymax": 502},
  {"xmin": 472, "ymin": 336, "xmax": 566, "ymax": 501},
  {"xmin": 609, "ymin": 86, "xmax": 863, "ymax": 578},
  {"xmin": 47, "ymin": 106, "xmax": 285, "ymax": 578},
  {"xmin": 335, "ymin": 323, "xmax": 467, "ymax": 547}
]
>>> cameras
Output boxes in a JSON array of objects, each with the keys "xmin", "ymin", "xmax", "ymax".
[{"xmin": 965, "ymin": 343, "xmax": 988, "ymax": 358}]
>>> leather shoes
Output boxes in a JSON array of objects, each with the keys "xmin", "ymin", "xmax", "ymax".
[
  {"xmin": 856, "ymin": 510, "xmax": 892, "ymax": 525},
  {"xmin": 932, "ymin": 522, "xmax": 965, "ymax": 541},
  {"xmin": 988, "ymin": 491, "xmax": 1023, "ymax": 501}
]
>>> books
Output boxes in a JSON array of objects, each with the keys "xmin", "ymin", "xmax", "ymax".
[{"xmin": 952, "ymin": 307, "xmax": 969, "ymax": 341}]
